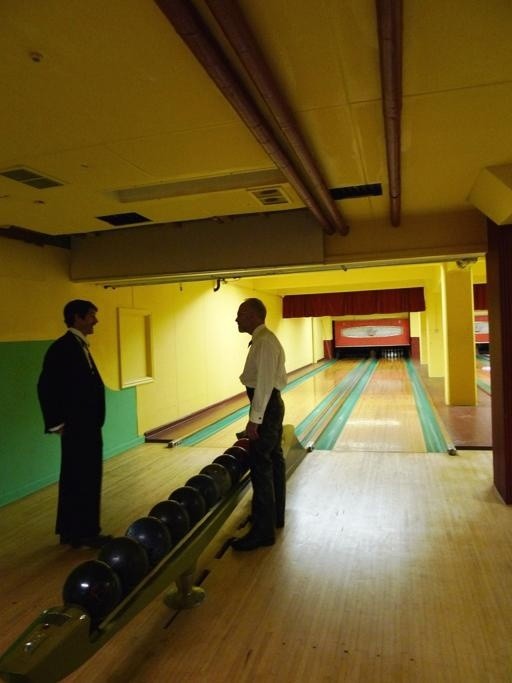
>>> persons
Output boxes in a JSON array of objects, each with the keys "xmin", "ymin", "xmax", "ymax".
[
  {"xmin": 235, "ymin": 298, "xmax": 286, "ymax": 551},
  {"xmin": 39, "ymin": 298, "xmax": 115, "ymax": 546}
]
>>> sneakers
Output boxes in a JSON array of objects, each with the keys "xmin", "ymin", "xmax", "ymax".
[
  {"xmin": 72, "ymin": 534, "xmax": 114, "ymax": 549},
  {"xmin": 230, "ymin": 529, "xmax": 276, "ymax": 551}
]
